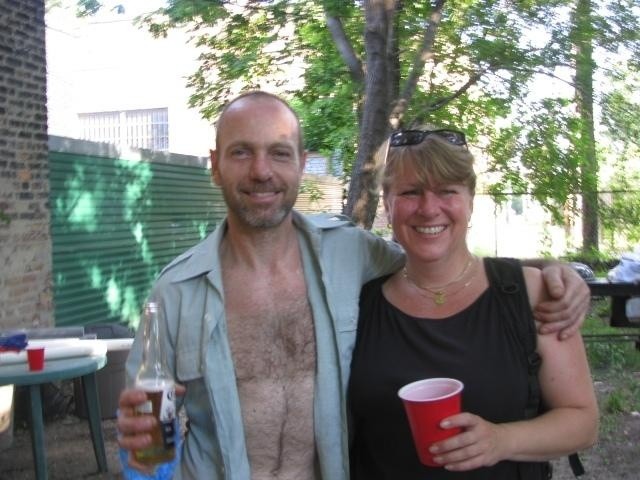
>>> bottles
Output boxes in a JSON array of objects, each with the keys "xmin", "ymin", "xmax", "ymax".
[{"xmin": 131, "ymin": 303, "xmax": 177, "ymax": 463}]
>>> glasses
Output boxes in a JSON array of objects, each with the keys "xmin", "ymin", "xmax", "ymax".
[{"xmin": 390, "ymin": 130, "xmax": 465, "ymax": 145}]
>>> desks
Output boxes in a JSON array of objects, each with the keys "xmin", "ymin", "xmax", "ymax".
[
  {"xmin": 588, "ymin": 276, "xmax": 640, "ymax": 327},
  {"xmin": 0, "ymin": 354, "xmax": 108, "ymax": 480}
]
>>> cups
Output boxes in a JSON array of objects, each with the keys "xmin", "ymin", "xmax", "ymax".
[
  {"xmin": 26, "ymin": 346, "xmax": 44, "ymax": 371},
  {"xmin": 396, "ymin": 377, "xmax": 465, "ymax": 468}
]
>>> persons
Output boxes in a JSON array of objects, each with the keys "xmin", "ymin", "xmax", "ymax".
[
  {"xmin": 117, "ymin": 88, "xmax": 592, "ymax": 480},
  {"xmin": 347, "ymin": 124, "xmax": 601, "ymax": 480}
]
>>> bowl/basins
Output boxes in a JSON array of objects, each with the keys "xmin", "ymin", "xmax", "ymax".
[{"xmin": 0, "ymin": 329, "xmax": 28, "ymax": 350}]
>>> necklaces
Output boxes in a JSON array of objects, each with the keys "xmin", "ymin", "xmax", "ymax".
[{"xmin": 400, "ymin": 257, "xmax": 479, "ymax": 305}]
